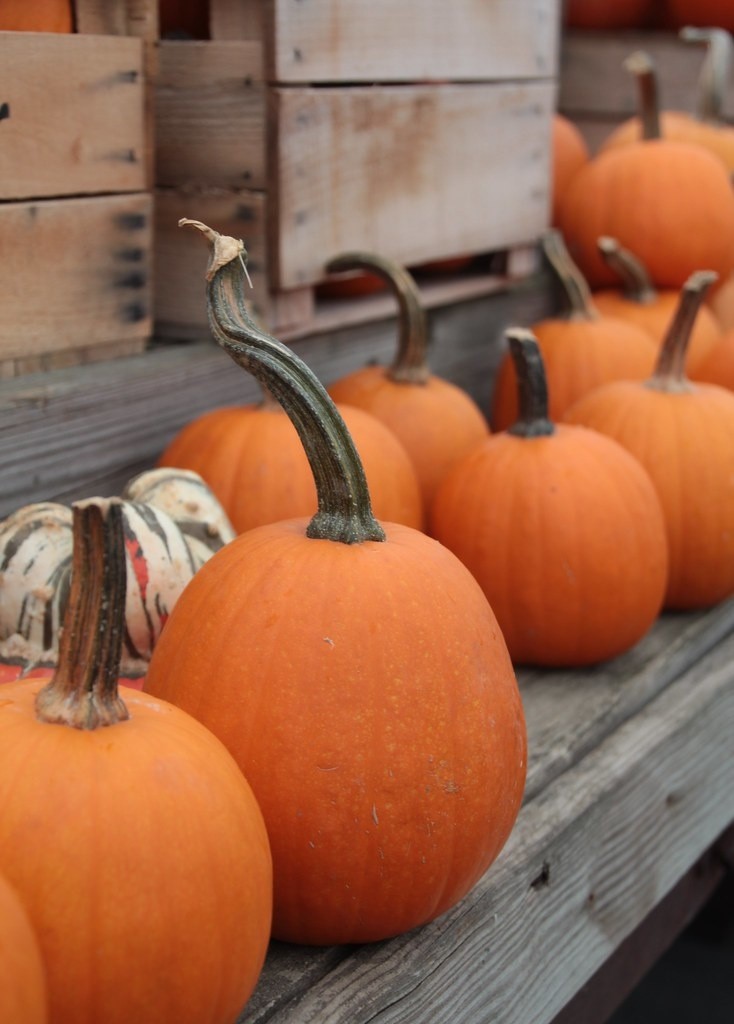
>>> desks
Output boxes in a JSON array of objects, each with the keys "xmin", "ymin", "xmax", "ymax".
[{"xmin": 0, "ymin": 383, "xmax": 734, "ymax": 1022}]
